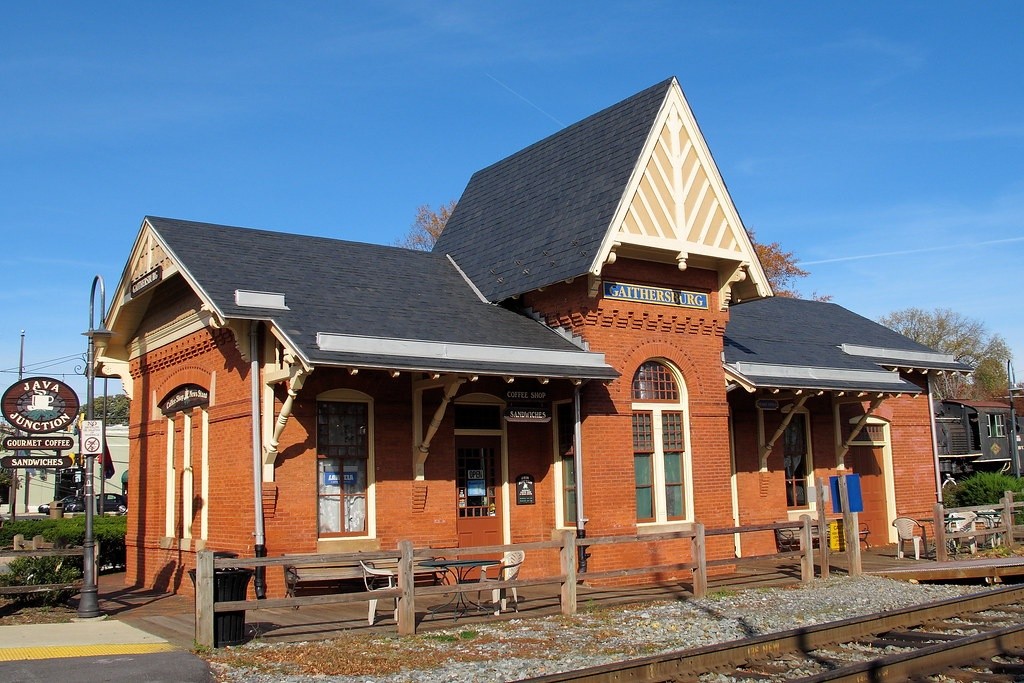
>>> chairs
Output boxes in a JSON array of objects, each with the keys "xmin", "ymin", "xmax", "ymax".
[
  {"xmin": 476, "ymin": 550, "xmax": 525, "ymax": 616},
  {"xmin": 947, "ymin": 510, "xmax": 978, "ymax": 554},
  {"xmin": 357, "ymin": 550, "xmax": 400, "ymax": 626},
  {"xmin": 892, "ymin": 516, "xmax": 928, "ymax": 561},
  {"xmin": 977, "ymin": 508, "xmax": 1003, "ymax": 548}
]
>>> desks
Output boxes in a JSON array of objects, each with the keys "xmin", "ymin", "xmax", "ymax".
[
  {"xmin": 911, "ymin": 518, "xmax": 966, "ymax": 557},
  {"xmin": 418, "ymin": 560, "xmax": 502, "ymax": 622},
  {"xmin": 972, "ymin": 510, "xmax": 1021, "ymax": 548}
]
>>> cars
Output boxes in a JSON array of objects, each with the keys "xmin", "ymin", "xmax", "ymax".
[
  {"xmin": 37, "ymin": 495, "xmax": 79, "ymax": 515},
  {"xmin": 67, "ymin": 493, "xmax": 128, "ymax": 515}
]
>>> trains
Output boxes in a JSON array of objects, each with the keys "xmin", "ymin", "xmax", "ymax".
[{"xmin": 935, "ymin": 399, "xmax": 1024, "ymax": 482}]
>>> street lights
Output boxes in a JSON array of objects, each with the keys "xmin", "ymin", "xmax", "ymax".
[
  {"xmin": 78, "ymin": 275, "xmax": 114, "ymax": 617},
  {"xmin": 1006, "ymin": 359, "xmax": 1023, "ymax": 478}
]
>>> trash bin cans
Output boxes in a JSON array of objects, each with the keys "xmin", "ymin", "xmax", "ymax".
[{"xmin": 187, "ymin": 551, "xmax": 255, "ymax": 646}]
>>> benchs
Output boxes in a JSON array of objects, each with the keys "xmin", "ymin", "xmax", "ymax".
[
  {"xmin": 773, "ymin": 517, "xmax": 872, "ymax": 554},
  {"xmin": 279, "ymin": 546, "xmax": 449, "ymax": 610}
]
